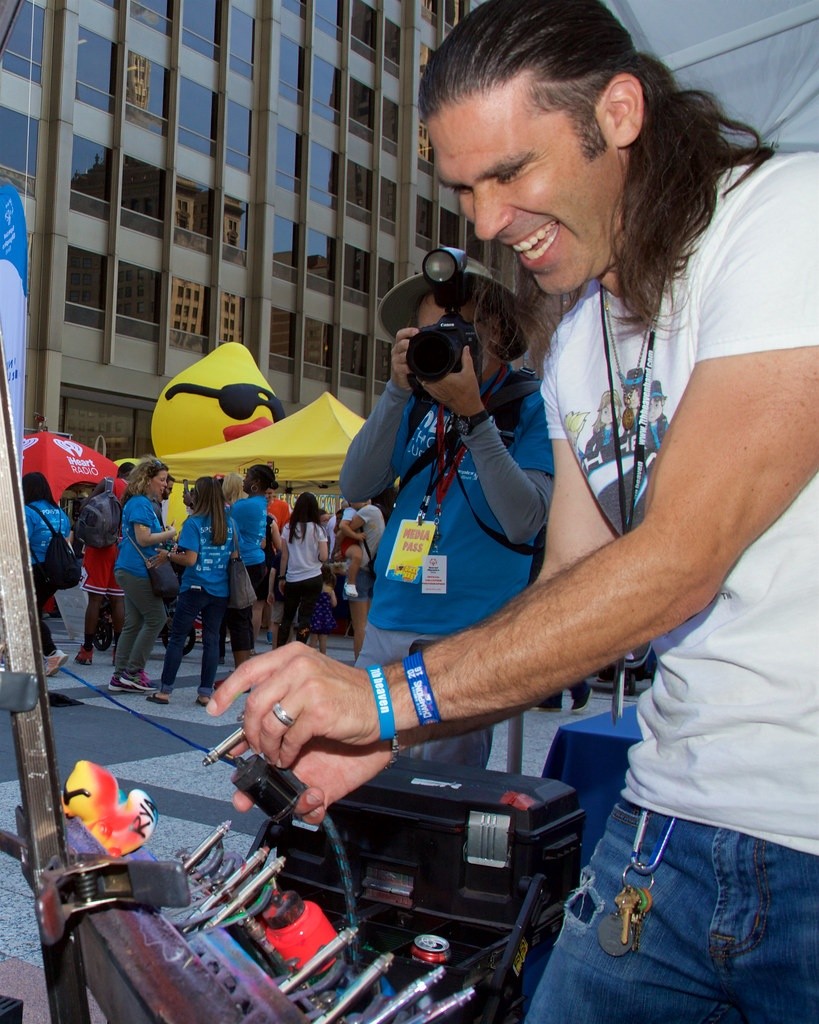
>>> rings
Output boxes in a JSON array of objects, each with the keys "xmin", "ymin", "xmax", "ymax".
[{"xmin": 273, "ymin": 703, "xmax": 294, "ymax": 727}]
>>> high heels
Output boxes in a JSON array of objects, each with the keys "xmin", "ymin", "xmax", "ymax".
[
  {"xmin": 75, "ymin": 644, "xmax": 94, "ymax": 665},
  {"xmin": 111, "ymin": 648, "xmax": 117, "ymax": 666}
]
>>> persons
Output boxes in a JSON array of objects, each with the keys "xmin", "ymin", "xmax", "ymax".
[
  {"xmin": 207, "ymin": 0, "xmax": 819, "ymax": 1024},
  {"xmin": 531, "ymin": 664, "xmax": 615, "ymax": 715},
  {"xmin": 24, "ymin": 456, "xmax": 387, "ymax": 706},
  {"xmin": 337, "ymin": 245, "xmax": 556, "ymax": 771}
]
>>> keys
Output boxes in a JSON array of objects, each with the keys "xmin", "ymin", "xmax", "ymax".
[{"xmin": 614, "ymin": 883, "xmax": 653, "ymax": 951}]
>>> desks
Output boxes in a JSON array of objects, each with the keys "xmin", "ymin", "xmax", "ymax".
[{"xmin": 543, "ymin": 704, "xmax": 644, "ymax": 869}]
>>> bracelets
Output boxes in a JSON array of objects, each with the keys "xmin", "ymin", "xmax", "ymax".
[
  {"xmin": 276, "ymin": 575, "xmax": 286, "ymax": 581},
  {"xmin": 366, "ymin": 666, "xmax": 397, "ymax": 740},
  {"xmin": 401, "ymin": 651, "xmax": 443, "ymax": 726}
]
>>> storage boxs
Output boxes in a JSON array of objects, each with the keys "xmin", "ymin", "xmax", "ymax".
[{"xmin": 266, "ymin": 754, "xmax": 588, "ymax": 1021}]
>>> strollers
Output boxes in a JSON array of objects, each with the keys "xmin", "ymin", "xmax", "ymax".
[{"xmin": 91, "ymin": 595, "xmax": 195, "ymax": 656}]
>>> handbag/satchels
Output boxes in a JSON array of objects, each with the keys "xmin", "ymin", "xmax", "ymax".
[
  {"xmin": 227, "ymin": 518, "xmax": 257, "ymax": 610},
  {"xmin": 147, "ymin": 560, "xmax": 179, "ymax": 599}
]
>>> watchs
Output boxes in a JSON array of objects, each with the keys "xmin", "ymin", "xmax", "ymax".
[
  {"xmin": 449, "ymin": 409, "xmax": 489, "ymax": 436},
  {"xmin": 166, "ymin": 551, "xmax": 172, "ymax": 563}
]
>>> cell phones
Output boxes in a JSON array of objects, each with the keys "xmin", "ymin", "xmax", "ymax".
[{"xmin": 183, "ymin": 479, "xmax": 189, "ymax": 493}]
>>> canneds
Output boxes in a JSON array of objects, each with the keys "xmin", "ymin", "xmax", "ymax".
[{"xmin": 411, "ymin": 933, "xmax": 451, "ymax": 965}]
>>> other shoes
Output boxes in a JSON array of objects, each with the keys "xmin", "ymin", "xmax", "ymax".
[
  {"xmin": 42, "ymin": 613, "xmax": 50, "ymax": 619},
  {"xmin": 596, "ymin": 678, "xmax": 613, "ymax": 684},
  {"xmin": 225, "ymin": 637, "xmax": 231, "ymax": 643},
  {"xmin": 219, "ymin": 656, "xmax": 225, "ymax": 664},
  {"xmin": 248, "ymin": 649, "xmax": 254, "ymax": 656}
]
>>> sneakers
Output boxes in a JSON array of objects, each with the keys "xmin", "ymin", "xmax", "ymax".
[
  {"xmin": 571, "ymin": 684, "xmax": 593, "ymax": 712},
  {"xmin": 265, "ymin": 631, "xmax": 273, "ymax": 645},
  {"xmin": 108, "ymin": 676, "xmax": 145, "ymax": 693},
  {"xmin": 119, "ymin": 668, "xmax": 158, "ymax": 691},
  {"xmin": 214, "ymin": 677, "xmax": 251, "ymax": 693},
  {"xmin": 46, "ymin": 650, "xmax": 69, "ymax": 677},
  {"xmin": 529, "ymin": 698, "xmax": 563, "ymax": 711}
]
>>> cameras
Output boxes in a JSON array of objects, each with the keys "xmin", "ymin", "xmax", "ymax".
[{"xmin": 406, "ymin": 247, "xmax": 480, "ymax": 381}]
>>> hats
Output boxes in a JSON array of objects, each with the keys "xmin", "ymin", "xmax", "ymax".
[
  {"xmin": 377, "ymin": 256, "xmax": 535, "ymax": 362},
  {"xmin": 117, "ymin": 462, "xmax": 136, "ymax": 475}
]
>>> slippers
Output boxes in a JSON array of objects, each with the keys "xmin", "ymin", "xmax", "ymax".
[
  {"xmin": 196, "ymin": 696, "xmax": 210, "ymax": 706},
  {"xmin": 146, "ymin": 692, "xmax": 169, "ymax": 704}
]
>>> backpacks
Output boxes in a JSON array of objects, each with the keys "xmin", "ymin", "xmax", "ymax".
[
  {"xmin": 360, "ymin": 503, "xmax": 391, "ymax": 583},
  {"xmin": 73, "ymin": 519, "xmax": 86, "ymax": 560},
  {"xmin": 26, "ymin": 504, "xmax": 82, "ymax": 590},
  {"xmin": 76, "ymin": 478, "xmax": 123, "ymax": 549}
]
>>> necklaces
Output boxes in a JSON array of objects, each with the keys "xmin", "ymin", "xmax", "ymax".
[{"xmin": 604, "ymin": 287, "xmax": 657, "ymax": 430}]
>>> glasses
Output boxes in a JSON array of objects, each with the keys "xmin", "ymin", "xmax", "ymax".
[
  {"xmin": 190, "ymin": 489, "xmax": 198, "ymax": 497},
  {"xmin": 151, "ymin": 461, "xmax": 161, "ymax": 471}
]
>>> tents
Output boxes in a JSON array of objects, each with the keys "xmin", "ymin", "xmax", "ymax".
[
  {"xmin": 21, "ymin": 431, "xmax": 121, "ymax": 505},
  {"xmin": 157, "ymin": 391, "xmax": 366, "ymax": 483}
]
